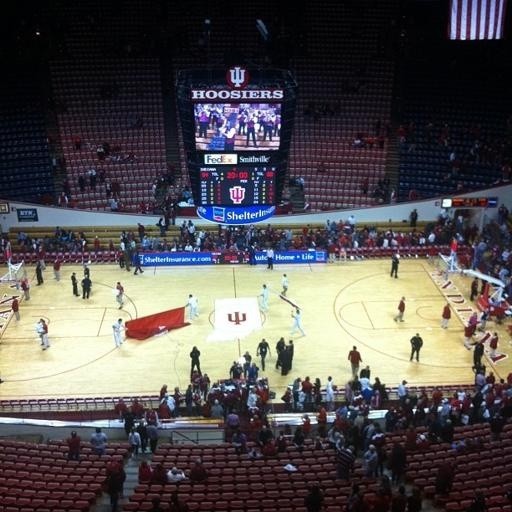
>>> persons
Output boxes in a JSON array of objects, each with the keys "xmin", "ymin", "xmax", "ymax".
[
  {"xmin": 196, "ymin": 221, "xmax": 329, "ymax": 337},
  {"xmin": 195, "ymin": 102, "xmax": 281, "ymax": 148},
  {"xmin": 323, "ymin": 201, "xmax": 512, "ymax": 371},
  {"xmin": 5, "ymin": 219, "xmax": 200, "ymax": 352},
  {"xmin": 39, "ymin": 132, "xmax": 199, "ymax": 238},
  {"xmin": 62, "ymin": 337, "xmax": 511, "ymax": 510},
  {"xmin": 288, "ymin": 102, "xmax": 503, "ymax": 203}
]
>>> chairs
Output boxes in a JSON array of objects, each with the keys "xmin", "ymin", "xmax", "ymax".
[
  {"xmin": 0, "ymin": 0, "xmax": 512, "ymax": 270},
  {"xmin": 0, "ymin": 382, "xmax": 512, "ymax": 512}
]
